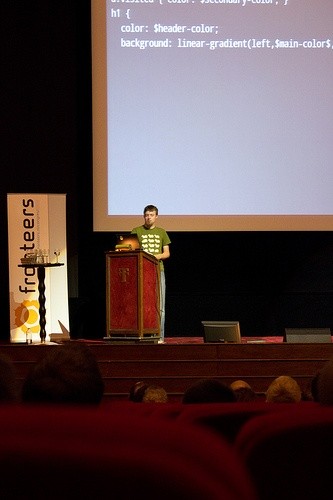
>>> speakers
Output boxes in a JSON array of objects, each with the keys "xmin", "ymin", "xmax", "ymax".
[{"xmin": 202, "ymin": 321, "xmax": 241, "ymax": 343}]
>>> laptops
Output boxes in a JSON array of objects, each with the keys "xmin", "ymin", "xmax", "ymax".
[{"xmin": 117, "ymin": 233, "xmax": 141, "ymax": 250}]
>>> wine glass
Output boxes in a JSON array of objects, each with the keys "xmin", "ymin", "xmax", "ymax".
[{"xmin": 54, "ymin": 249, "xmax": 60, "ymax": 262}]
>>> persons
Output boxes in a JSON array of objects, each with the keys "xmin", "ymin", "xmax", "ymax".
[
  {"xmin": 128, "ymin": 205, "xmax": 170, "ymax": 344},
  {"xmin": 22, "ymin": 347, "xmax": 333, "ymax": 405}
]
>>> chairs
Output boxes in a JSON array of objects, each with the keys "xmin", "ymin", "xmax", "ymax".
[
  {"xmin": 178, "ymin": 401, "xmax": 273, "ymax": 446},
  {"xmin": 0, "ymin": 404, "xmax": 255, "ymax": 500},
  {"xmin": 234, "ymin": 406, "xmax": 333, "ymax": 500}
]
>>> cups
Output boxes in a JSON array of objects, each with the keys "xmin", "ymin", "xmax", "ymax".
[{"xmin": 35, "ymin": 248, "xmax": 50, "ymax": 263}]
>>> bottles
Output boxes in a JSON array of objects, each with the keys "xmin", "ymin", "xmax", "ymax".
[{"xmin": 26, "ymin": 328, "xmax": 32, "ymax": 344}]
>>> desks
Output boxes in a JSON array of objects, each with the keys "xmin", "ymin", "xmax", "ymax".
[{"xmin": 18, "ymin": 262, "xmax": 64, "ymax": 346}]
